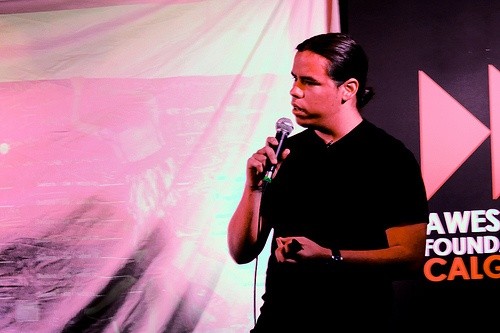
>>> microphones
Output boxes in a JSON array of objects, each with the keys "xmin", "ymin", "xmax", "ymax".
[{"xmin": 261, "ymin": 118, "xmax": 293, "ymax": 188}]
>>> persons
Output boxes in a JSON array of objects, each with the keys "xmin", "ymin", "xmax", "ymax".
[{"xmin": 226, "ymin": 32, "xmax": 430, "ymax": 333}]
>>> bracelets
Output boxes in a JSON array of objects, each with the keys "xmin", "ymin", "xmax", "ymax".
[{"xmin": 327, "ymin": 245, "xmax": 343, "ymax": 265}]
